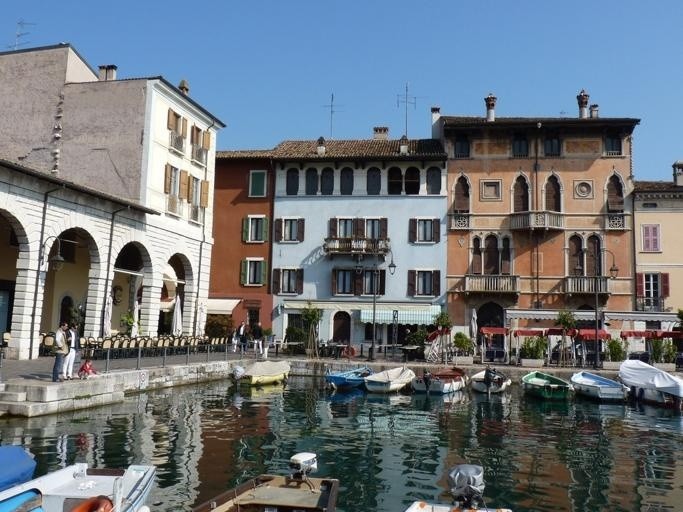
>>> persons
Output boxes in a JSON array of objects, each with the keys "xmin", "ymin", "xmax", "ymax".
[
  {"xmin": 551, "ymin": 339, "xmax": 562, "ymax": 353},
  {"xmin": 250, "ymin": 321, "xmax": 263, "ymax": 357},
  {"xmin": 236, "ymin": 321, "xmax": 248, "ymax": 352},
  {"xmin": 239, "ymin": 332, "xmax": 247, "ymax": 355},
  {"xmin": 229, "ymin": 327, "xmax": 239, "ymax": 353},
  {"xmin": 61, "ymin": 322, "xmax": 79, "ymax": 381},
  {"xmin": 50, "ymin": 321, "xmax": 69, "ymax": 383},
  {"xmin": 401, "ymin": 328, "xmax": 414, "ymax": 358},
  {"xmin": 76, "ymin": 358, "xmax": 97, "ymax": 381}
]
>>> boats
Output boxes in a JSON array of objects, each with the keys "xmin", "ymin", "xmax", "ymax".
[
  {"xmin": 523, "ymin": 370, "xmax": 575, "ymax": 400},
  {"xmin": 570, "ymin": 370, "xmax": 629, "ymax": 403},
  {"xmin": 364, "ymin": 365, "xmax": 415, "ymax": 394},
  {"xmin": 620, "ymin": 360, "xmax": 683, "ymax": 413},
  {"xmin": 411, "ymin": 365, "xmax": 470, "ymax": 394},
  {"xmin": 238, "ymin": 360, "xmax": 291, "ymax": 386},
  {"xmin": 323, "ymin": 365, "xmax": 371, "ymax": 390},
  {"xmin": 471, "ymin": 366, "xmax": 511, "ymax": 397}
]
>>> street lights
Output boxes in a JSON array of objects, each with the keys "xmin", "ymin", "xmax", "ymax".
[
  {"xmin": 575, "ymin": 240, "xmax": 618, "ymax": 369},
  {"xmin": 357, "ymin": 245, "xmax": 396, "ymax": 362},
  {"xmin": 28, "ymin": 234, "xmax": 64, "ymax": 359}
]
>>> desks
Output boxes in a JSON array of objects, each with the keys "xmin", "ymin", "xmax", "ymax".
[{"xmin": 249, "ymin": 339, "xmax": 420, "ymax": 362}]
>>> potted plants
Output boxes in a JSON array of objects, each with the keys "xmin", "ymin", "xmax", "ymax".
[{"xmin": 301, "ymin": 299, "xmax": 323, "ymax": 355}]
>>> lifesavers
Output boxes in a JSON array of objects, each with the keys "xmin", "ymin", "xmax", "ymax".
[{"xmin": 71, "ymin": 497, "xmax": 113, "ymax": 512}]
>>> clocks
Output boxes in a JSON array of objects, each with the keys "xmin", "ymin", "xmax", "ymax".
[{"xmin": 113, "ymin": 285, "xmax": 123, "ymax": 305}]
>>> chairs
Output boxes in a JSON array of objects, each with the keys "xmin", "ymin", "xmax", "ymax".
[{"xmin": 3, "ymin": 333, "xmax": 229, "ymax": 360}]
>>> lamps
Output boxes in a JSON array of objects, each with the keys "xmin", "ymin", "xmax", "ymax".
[{"xmin": 42, "ymin": 236, "xmax": 64, "ymax": 271}]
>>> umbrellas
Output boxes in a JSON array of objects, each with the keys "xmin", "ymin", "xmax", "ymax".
[
  {"xmin": 103, "ymin": 296, "xmax": 112, "ymax": 338},
  {"xmin": 128, "ymin": 300, "xmax": 139, "ymax": 339},
  {"xmin": 468, "ymin": 308, "xmax": 478, "ymax": 348},
  {"xmin": 169, "ymin": 294, "xmax": 183, "ymax": 340}
]
